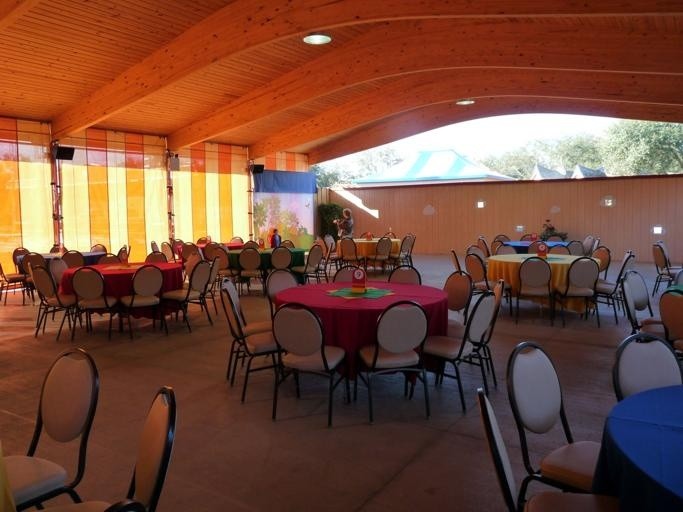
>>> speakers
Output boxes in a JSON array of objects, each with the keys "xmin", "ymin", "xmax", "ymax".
[
  {"xmin": 250, "ymin": 164, "xmax": 264, "ymax": 174},
  {"xmin": 52, "ymin": 146, "xmax": 74, "ymax": 160}
]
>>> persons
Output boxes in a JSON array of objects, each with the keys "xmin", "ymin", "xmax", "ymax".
[
  {"xmin": 332, "ymin": 208, "xmax": 355, "ymax": 240},
  {"xmin": 270, "ymin": 228, "xmax": 282, "ymax": 249}
]
